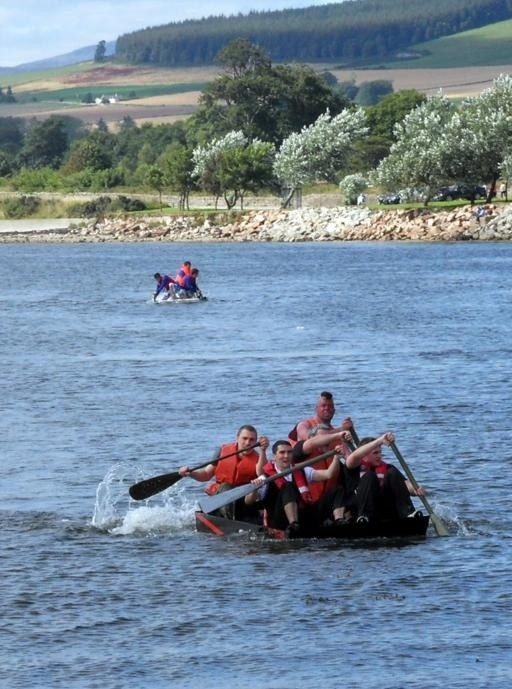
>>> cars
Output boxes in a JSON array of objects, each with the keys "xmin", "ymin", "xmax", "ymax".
[{"xmin": 378, "ymin": 183, "xmax": 487, "ymax": 204}]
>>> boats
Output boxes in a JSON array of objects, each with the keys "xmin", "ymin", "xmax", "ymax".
[
  {"xmin": 153, "ymin": 291, "xmax": 200, "ymax": 304},
  {"xmin": 195, "ymin": 498, "xmax": 430, "ymax": 539}
]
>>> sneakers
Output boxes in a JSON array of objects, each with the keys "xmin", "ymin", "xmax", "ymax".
[{"xmin": 284, "ymin": 513, "xmax": 371, "ymax": 536}]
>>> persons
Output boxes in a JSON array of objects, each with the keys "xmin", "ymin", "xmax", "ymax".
[
  {"xmin": 483, "ymin": 182, "xmax": 488, "ymax": 199},
  {"xmin": 500, "ymin": 182, "xmax": 508, "ymax": 200},
  {"xmin": 178, "ymin": 391, "xmax": 426, "ymax": 528},
  {"xmin": 473, "ymin": 205, "xmax": 498, "ymax": 221},
  {"xmin": 357, "ymin": 193, "xmax": 365, "ymax": 205},
  {"xmin": 152, "ymin": 261, "xmax": 202, "ymax": 301}
]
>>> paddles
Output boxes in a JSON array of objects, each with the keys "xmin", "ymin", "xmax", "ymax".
[
  {"xmin": 129, "ymin": 442, "xmax": 260, "ymax": 500},
  {"xmin": 197, "ymin": 448, "xmax": 340, "ymax": 515},
  {"xmin": 391, "ymin": 441, "xmax": 450, "ymax": 536}
]
